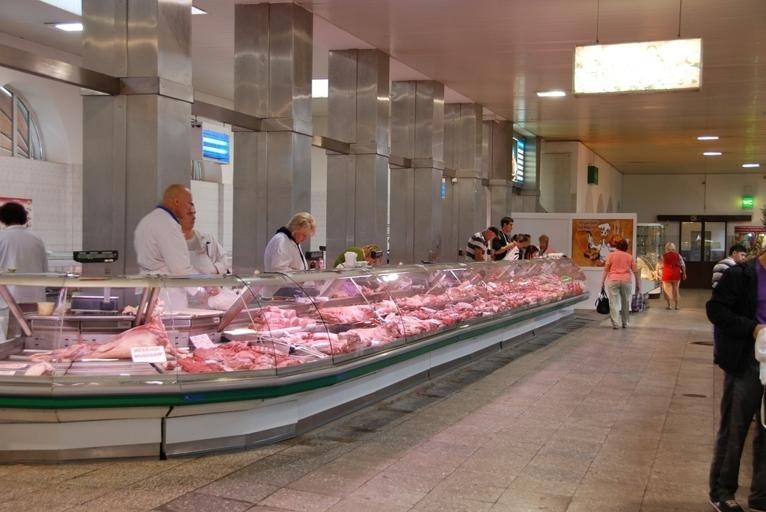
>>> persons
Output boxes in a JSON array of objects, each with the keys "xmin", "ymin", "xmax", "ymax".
[
  {"xmin": 711, "ymin": 244, "xmax": 746, "ymax": 363},
  {"xmin": 600, "ymin": 239, "xmax": 642, "ymax": 329},
  {"xmin": 264, "ymin": 211, "xmax": 315, "ymax": 272},
  {"xmin": 706, "ymin": 250, "xmax": 766, "ymax": 512},
  {"xmin": 133, "ymin": 185, "xmax": 194, "ymax": 278},
  {"xmin": 0, "ymin": 203, "xmax": 48, "ymax": 274},
  {"xmin": 661, "ymin": 242, "xmax": 686, "ymax": 311},
  {"xmin": 333, "ymin": 244, "xmax": 383, "ymax": 268},
  {"xmin": 177, "ymin": 202, "xmax": 228, "ymax": 276},
  {"xmin": 465, "ymin": 217, "xmax": 555, "ymax": 262}
]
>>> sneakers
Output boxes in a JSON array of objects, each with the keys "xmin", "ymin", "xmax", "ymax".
[{"xmin": 709, "ymin": 498, "xmax": 744, "ymax": 512}]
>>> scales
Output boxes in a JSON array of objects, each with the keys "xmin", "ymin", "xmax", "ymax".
[
  {"xmin": 69, "ymin": 251, "xmax": 119, "ymax": 320},
  {"xmin": 304, "ymin": 246, "xmax": 325, "ymax": 289}
]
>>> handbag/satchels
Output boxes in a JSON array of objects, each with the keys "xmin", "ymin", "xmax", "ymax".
[
  {"xmin": 595, "ymin": 288, "xmax": 609, "ymax": 314},
  {"xmin": 631, "ymin": 294, "xmax": 642, "ymax": 311}
]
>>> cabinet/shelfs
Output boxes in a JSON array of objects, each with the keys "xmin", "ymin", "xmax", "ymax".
[{"xmin": 0, "ymin": 251, "xmax": 591, "ymax": 459}]
